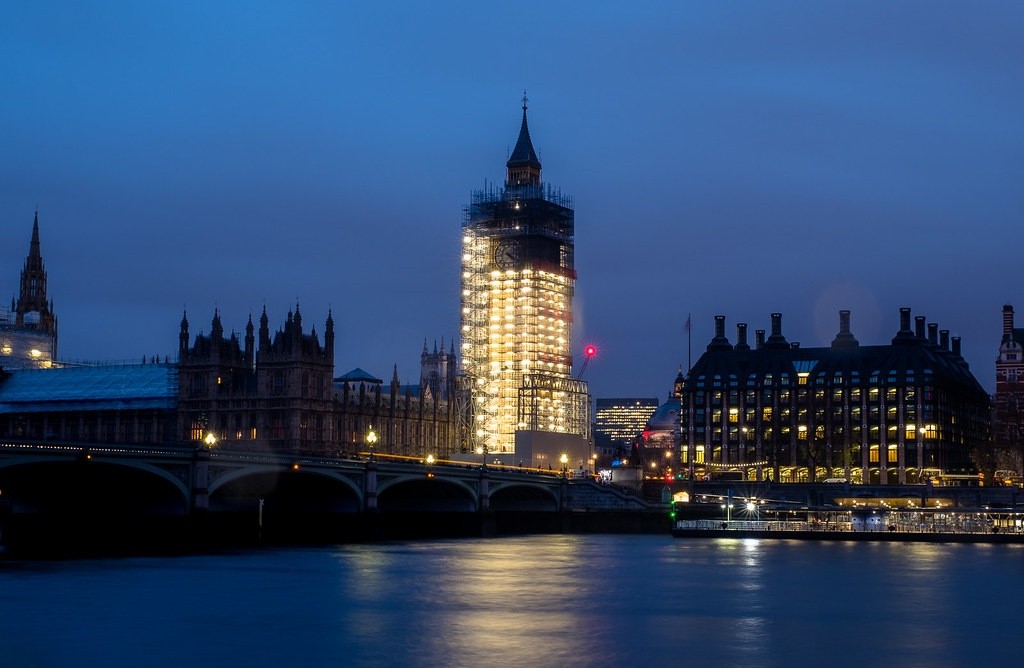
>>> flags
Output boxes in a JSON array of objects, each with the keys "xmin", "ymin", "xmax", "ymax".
[{"xmin": 684, "ymin": 314, "xmax": 691, "ymax": 331}]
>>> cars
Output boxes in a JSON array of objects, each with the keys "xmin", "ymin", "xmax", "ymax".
[{"xmin": 823, "ymin": 477, "xmax": 847, "ymax": 484}]
